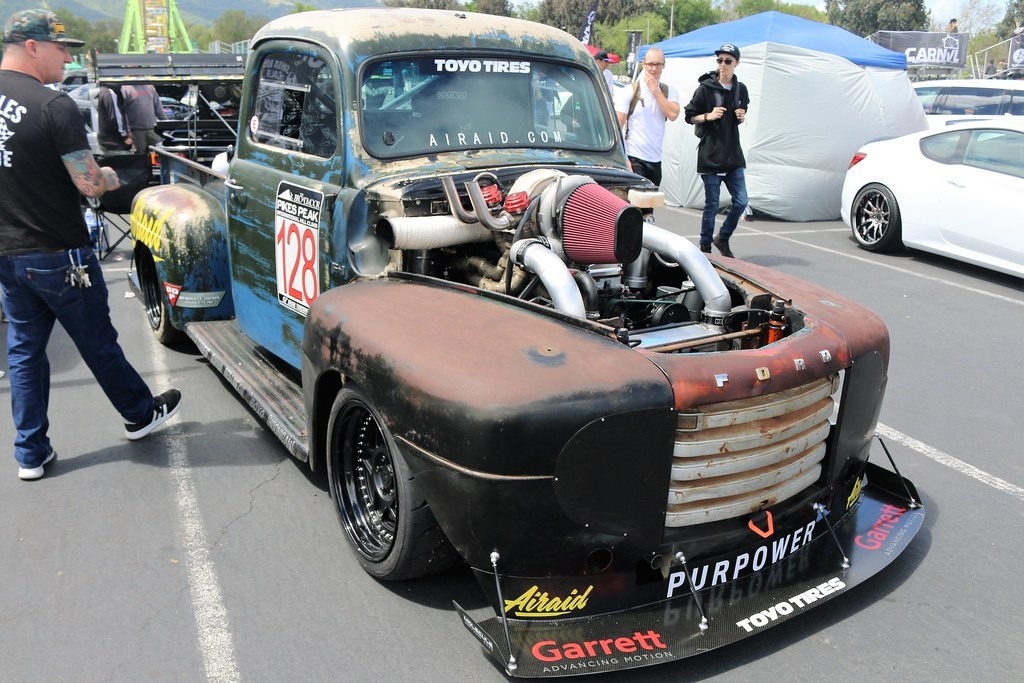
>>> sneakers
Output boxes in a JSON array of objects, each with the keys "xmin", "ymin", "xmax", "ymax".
[
  {"xmin": 18, "ymin": 445, "xmax": 55, "ymax": 479},
  {"xmin": 124, "ymin": 388, "xmax": 182, "ymax": 440}
]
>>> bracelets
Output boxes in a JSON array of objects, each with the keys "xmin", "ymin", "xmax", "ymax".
[{"xmin": 704, "ymin": 113, "xmax": 707, "ymax": 121}]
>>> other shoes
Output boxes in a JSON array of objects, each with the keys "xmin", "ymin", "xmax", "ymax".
[
  {"xmin": 700, "ymin": 242, "xmax": 711, "ymax": 253},
  {"xmin": 713, "ymin": 234, "xmax": 734, "ymax": 258}
]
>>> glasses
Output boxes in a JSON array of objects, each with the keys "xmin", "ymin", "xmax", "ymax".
[
  {"xmin": 716, "ymin": 58, "xmax": 732, "ymax": 65},
  {"xmin": 644, "ymin": 63, "xmax": 665, "ymax": 68}
]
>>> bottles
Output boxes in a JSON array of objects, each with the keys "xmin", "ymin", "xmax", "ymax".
[{"xmin": 85, "ymin": 208, "xmax": 94, "ymax": 225}]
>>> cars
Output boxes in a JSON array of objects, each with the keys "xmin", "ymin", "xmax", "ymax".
[
  {"xmin": 48, "ymin": 49, "xmax": 313, "ymax": 233},
  {"xmin": 125, "ymin": 5, "xmax": 928, "ymax": 682},
  {"xmin": 840, "ymin": 115, "xmax": 1024, "ymax": 284},
  {"xmin": 910, "ymin": 77, "xmax": 1024, "ymax": 152}
]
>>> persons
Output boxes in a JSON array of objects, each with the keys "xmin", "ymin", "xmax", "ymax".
[
  {"xmin": 613, "ymin": 47, "xmax": 680, "ymax": 215},
  {"xmin": 560, "ymin": 94, "xmax": 588, "ymax": 146},
  {"xmin": 97, "ymin": 64, "xmax": 168, "ymax": 153},
  {"xmin": 985, "ymin": 59, "xmax": 1007, "ymax": 79},
  {"xmin": 945, "ymin": 19, "xmax": 958, "ymax": 74},
  {"xmin": 0, "ymin": 8, "xmax": 181, "ymax": 480},
  {"xmin": 594, "ymin": 50, "xmax": 613, "ymax": 96},
  {"xmin": 684, "ymin": 43, "xmax": 750, "ymax": 258}
]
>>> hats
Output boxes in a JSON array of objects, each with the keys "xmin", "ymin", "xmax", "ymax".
[
  {"xmin": 715, "ymin": 44, "xmax": 740, "ymax": 60},
  {"xmin": 3, "ymin": 8, "xmax": 86, "ymax": 48},
  {"xmin": 594, "ymin": 51, "xmax": 611, "ymax": 61}
]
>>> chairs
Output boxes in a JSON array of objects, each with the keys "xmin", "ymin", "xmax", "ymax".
[{"xmin": 85, "ymin": 151, "xmax": 161, "ymax": 261}]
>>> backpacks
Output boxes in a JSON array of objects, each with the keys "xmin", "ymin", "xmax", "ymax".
[{"xmin": 694, "ymin": 81, "xmax": 740, "ymax": 139}]
